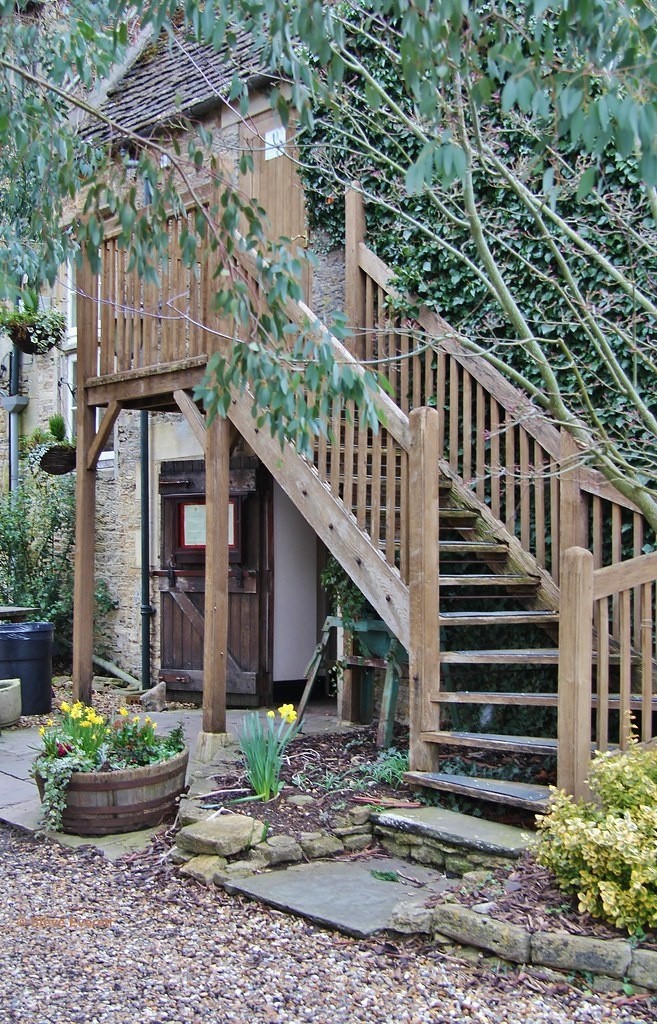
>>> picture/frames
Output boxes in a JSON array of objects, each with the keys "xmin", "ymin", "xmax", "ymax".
[{"xmin": 179, "ymin": 500, "xmax": 237, "ymax": 548}]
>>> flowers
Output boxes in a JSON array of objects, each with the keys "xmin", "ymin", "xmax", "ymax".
[
  {"xmin": 0, "ymin": 295, "xmax": 69, "ymax": 354},
  {"xmin": 30, "ymin": 699, "xmax": 186, "ymax": 833},
  {"xmin": 19, "ymin": 426, "xmax": 77, "ymax": 475}
]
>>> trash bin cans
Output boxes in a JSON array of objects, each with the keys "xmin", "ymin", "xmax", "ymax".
[{"xmin": 0, "ymin": 622, "xmax": 56, "ymax": 715}]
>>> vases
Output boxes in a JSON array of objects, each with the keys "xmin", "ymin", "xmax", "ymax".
[
  {"xmin": 39, "ymin": 445, "xmax": 77, "ymax": 475},
  {"xmin": 33, "ymin": 735, "xmax": 189, "ymax": 834},
  {"xmin": 6, "ymin": 324, "xmax": 63, "ymax": 355}
]
